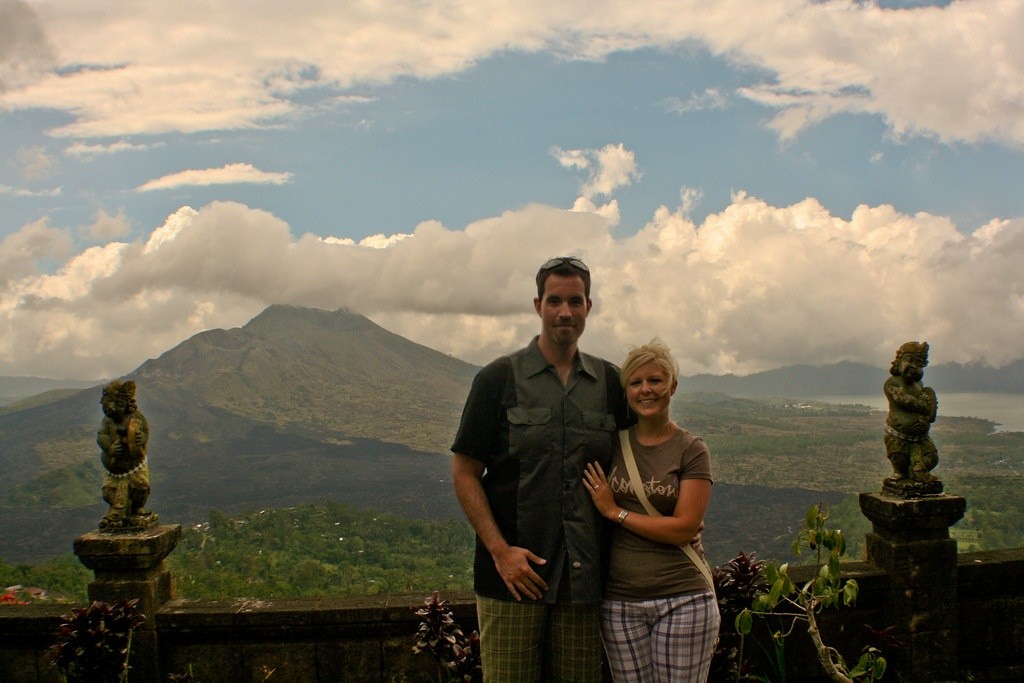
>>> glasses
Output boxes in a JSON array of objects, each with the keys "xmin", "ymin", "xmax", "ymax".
[{"xmin": 539, "ymin": 259, "xmax": 590, "ymax": 277}]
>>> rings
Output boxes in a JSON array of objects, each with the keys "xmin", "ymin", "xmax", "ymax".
[{"xmin": 594, "ymin": 485, "xmax": 599, "ymax": 489}]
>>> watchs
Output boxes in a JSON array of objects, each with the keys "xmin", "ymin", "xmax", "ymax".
[{"xmin": 617, "ymin": 509, "xmax": 629, "ymax": 524}]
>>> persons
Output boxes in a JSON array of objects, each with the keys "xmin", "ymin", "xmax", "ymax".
[
  {"xmin": 884, "ymin": 342, "xmax": 939, "ymax": 482},
  {"xmin": 583, "ymin": 337, "xmax": 721, "ymax": 683},
  {"xmin": 450, "ymin": 257, "xmax": 706, "ymax": 683},
  {"xmin": 97, "ymin": 378, "xmax": 151, "ymax": 515}
]
